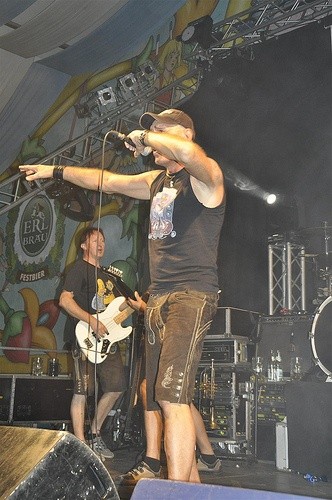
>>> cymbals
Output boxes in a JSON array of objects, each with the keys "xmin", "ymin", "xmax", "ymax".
[
  {"xmin": 293, "ymin": 226, "xmax": 332, "ymax": 248},
  {"xmin": 317, "ymin": 252, "xmax": 332, "ymax": 268}
]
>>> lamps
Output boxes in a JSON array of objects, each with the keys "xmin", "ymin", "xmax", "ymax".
[
  {"xmin": 73, "ymin": 97, "xmax": 96, "ymax": 118},
  {"xmin": 119, "ymin": 72, "xmax": 138, "ymax": 91},
  {"xmin": 97, "ymin": 87, "xmax": 117, "ymax": 105},
  {"xmin": 180, "ymin": 15, "xmax": 212, "ymax": 48},
  {"xmin": 138, "ymin": 60, "xmax": 160, "ymax": 83}
]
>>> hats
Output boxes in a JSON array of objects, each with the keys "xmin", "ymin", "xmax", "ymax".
[{"xmin": 138, "ymin": 108, "xmax": 197, "ymax": 141}]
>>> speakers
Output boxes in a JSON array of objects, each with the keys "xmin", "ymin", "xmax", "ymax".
[
  {"xmin": 0, "ymin": 426, "xmax": 121, "ymax": 500},
  {"xmin": 129, "ymin": 477, "xmax": 329, "ymax": 500},
  {"xmin": 255, "ymin": 315, "xmax": 316, "ymax": 375}
]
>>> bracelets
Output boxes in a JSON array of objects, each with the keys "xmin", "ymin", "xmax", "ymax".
[
  {"xmin": 53, "ymin": 165, "xmax": 67, "ymax": 180},
  {"xmin": 139, "ymin": 129, "xmax": 149, "ymax": 147}
]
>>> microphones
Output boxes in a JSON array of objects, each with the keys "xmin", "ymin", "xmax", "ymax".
[{"xmin": 110, "ymin": 130, "xmax": 152, "ymax": 156}]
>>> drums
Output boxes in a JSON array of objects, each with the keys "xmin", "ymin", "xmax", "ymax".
[{"xmin": 308, "ymin": 295, "xmax": 332, "ymax": 381}]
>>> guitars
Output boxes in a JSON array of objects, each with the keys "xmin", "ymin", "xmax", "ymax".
[
  {"xmin": 100, "ymin": 264, "xmax": 148, "ymax": 313},
  {"xmin": 74, "ymin": 288, "xmax": 151, "ymax": 364}
]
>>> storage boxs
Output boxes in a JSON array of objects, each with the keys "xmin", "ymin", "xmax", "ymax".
[
  {"xmin": 0, "ymin": 374, "xmax": 76, "ymax": 424},
  {"xmin": 203, "ymin": 307, "xmax": 264, "ymax": 338},
  {"xmin": 275, "ymin": 423, "xmax": 291, "ymax": 471}
]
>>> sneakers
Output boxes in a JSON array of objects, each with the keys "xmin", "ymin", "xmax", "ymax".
[
  {"xmin": 85, "ymin": 435, "xmax": 115, "ymax": 459},
  {"xmin": 81, "ymin": 441, "xmax": 105, "ymax": 462},
  {"xmin": 195, "ymin": 454, "xmax": 223, "ymax": 473},
  {"xmin": 116, "ymin": 460, "xmax": 164, "ymax": 486}
]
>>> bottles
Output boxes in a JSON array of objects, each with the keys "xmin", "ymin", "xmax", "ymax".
[
  {"xmin": 113, "ymin": 408, "xmax": 122, "ymax": 443},
  {"xmin": 268, "ymin": 350, "xmax": 282, "ymax": 382}
]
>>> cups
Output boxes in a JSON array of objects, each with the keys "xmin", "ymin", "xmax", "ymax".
[
  {"xmin": 289, "ymin": 356, "xmax": 302, "ymax": 383},
  {"xmin": 31, "ymin": 356, "xmax": 44, "ymax": 376},
  {"xmin": 47, "ymin": 358, "xmax": 59, "ymax": 376},
  {"xmin": 252, "ymin": 356, "xmax": 264, "ymax": 375}
]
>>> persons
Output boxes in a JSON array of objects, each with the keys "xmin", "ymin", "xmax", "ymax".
[
  {"xmin": 60, "ymin": 227, "xmax": 129, "ymax": 461},
  {"xmin": 18, "ymin": 109, "xmax": 226, "ymax": 485},
  {"xmin": 120, "ymin": 291, "xmax": 223, "ymax": 483}
]
